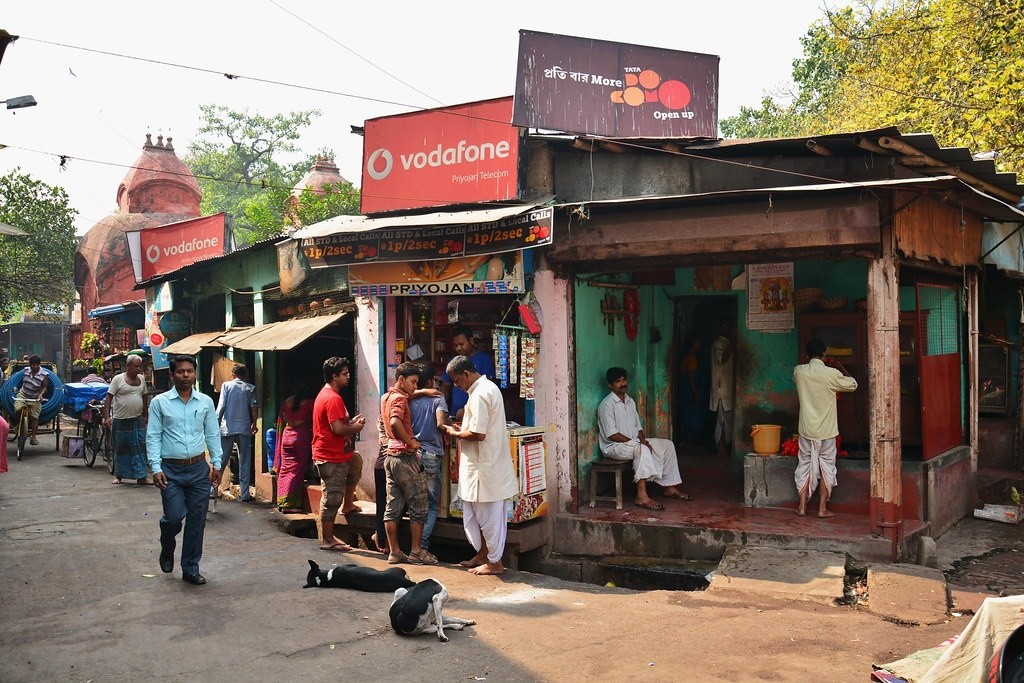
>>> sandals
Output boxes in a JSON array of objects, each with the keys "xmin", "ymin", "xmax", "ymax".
[
  {"xmin": 388, "ymin": 551, "xmax": 408, "ymax": 564},
  {"xmin": 408, "ymin": 548, "xmax": 438, "ymax": 565}
]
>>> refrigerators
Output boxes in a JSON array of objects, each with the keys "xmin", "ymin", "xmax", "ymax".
[{"xmin": 447, "ymin": 427, "xmax": 548, "ymax": 524}]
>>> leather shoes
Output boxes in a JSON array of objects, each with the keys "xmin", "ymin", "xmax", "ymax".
[
  {"xmin": 183, "ymin": 571, "xmax": 206, "ymax": 585},
  {"xmin": 159, "ymin": 552, "xmax": 174, "ymax": 573}
]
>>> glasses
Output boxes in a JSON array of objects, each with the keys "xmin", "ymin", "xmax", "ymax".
[{"xmin": 336, "ymin": 372, "xmax": 350, "ymax": 378}]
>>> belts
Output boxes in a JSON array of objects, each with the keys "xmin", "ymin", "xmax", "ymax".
[{"xmin": 163, "ymin": 453, "xmax": 205, "ymax": 466}]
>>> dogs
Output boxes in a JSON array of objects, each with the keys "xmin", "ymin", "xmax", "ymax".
[
  {"xmin": 302, "ymin": 560, "xmax": 416, "ymax": 593},
  {"xmin": 388, "ymin": 577, "xmax": 477, "ymax": 642}
]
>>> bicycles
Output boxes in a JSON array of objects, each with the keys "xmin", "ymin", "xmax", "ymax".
[{"xmin": 82, "ymin": 403, "xmax": 114, "ymax": 473}]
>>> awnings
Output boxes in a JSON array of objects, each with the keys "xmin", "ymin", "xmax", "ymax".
[
  {"xmin": 160, "ymin": 313, "xmax": 350, "ymax": 356},
  {"xmin": 291, "ymin": 201, "xmax": 547, "ymax": 242},
  {"xmin": 553, "ymin": 173, "xmax": 1024, "ymax": 221},
  {"xmin": 132, "ymin": 236, "xmax": 290, "ymax": 291}
]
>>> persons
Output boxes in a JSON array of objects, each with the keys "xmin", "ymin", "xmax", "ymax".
[
  {"xmin": 1, "ymin": 353, "xmax": 109, "ymax": 445},
  {"xmin": 208, "ymin": 362, "xmax": 315, "ymax": 513},
  {"xmin": 143, "ymin": 355, "xmax": 224, "ymax": 586},
  {"xmin": 597, "ymin": 367, "xmax": 692, "ymax": 511},
  {"xmin": 372, "ymin": 327, "xmax": 520, "ymax": 575},
  {"xmin": 678, "ymin": 318, "xmax": 733, "ymax": 456},
  {"xmin": 311, "ymin": 357, "xmax": 367, "ymax": 551},
  {"xmin": 792, "ymin": 339, "xmax": 858, "ymax": 518},
  {"xmin": 103, "ymin": 354, "xmax": 155, "ymax": 485}
]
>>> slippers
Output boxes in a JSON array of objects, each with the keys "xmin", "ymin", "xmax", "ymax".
[
  {"xmin": 636, "ymin": 497, "xmax": 665, "ymax": 512},
  {"xmin": 338, "ymin": 504, "xmax": 363, "ymax": 515},
  {"xmin": 319, "ymin": 541, "xmax": 352, "ymax": 552},
  {"xmin": 666, "ymin": 490, "xmax": 693, "ymax": 502}
]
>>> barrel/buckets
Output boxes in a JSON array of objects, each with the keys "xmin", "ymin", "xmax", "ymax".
[{"xmin": 751, "ymin": 424, "xmax": 781, "ymax": 455}]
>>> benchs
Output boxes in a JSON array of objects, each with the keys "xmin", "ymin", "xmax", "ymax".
[{"xmin": 590, "ymin": 456, "xmax": 634, "ymax": 510}]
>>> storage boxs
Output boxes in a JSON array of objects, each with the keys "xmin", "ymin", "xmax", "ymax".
[{"xmin": 62, "ymin": 435, "xmax": 85, "ymax": 458}]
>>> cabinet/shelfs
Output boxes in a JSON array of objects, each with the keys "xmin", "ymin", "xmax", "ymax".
[
  {"xmin": 799, "ymin": 310, "xmax": 929, "ymax": 446},
  {"xmin": 385, "ymin": 296, "xmax": 493, "ymax": 392}
]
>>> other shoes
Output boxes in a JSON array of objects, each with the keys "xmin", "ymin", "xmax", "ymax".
[
  {"xmin": 30, "ymin": 438, "xmax": 39, "ymax": 445},
  {"xmin": 242, "ymin": 495, "xmax": 257, "ymax": 503},
  {"xmin": 14, "ymin": 424, "xmax": 20, "ymax": 434},
  {"xmin": 278, "ymin": 506, "xmax": 304, "ymax": 513}
]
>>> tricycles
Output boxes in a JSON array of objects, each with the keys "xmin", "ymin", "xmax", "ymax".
[{"xmin": 6, "ymin": 395, "xmax": 63, "ymax": 461}]
